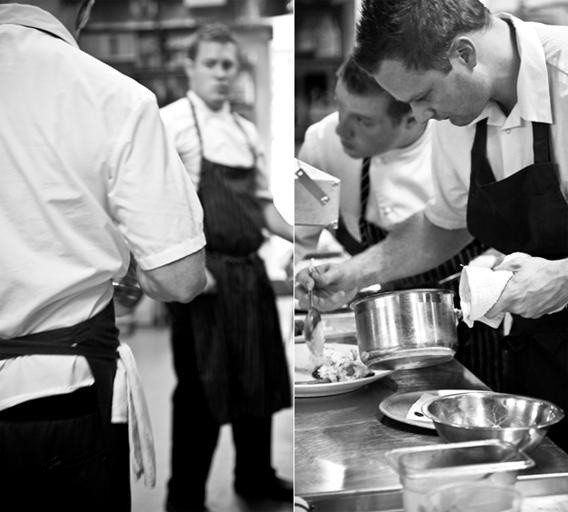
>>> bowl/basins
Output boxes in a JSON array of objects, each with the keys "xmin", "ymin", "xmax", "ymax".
[{"xmin": 422, "ymin": 391, "xmax": 566, "ymax": 457}]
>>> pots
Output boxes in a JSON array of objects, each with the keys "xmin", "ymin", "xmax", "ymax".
[{"xmin": 350, "ymin": 266, "xmax": 462, "ymax": 374}]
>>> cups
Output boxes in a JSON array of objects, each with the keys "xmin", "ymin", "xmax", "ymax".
[{"xmin": 429, "ymin": 485, "xmax": 524, "ymax": 511}]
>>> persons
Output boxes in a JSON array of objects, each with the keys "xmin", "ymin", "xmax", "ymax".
[
  {"xmin": 1, "ymin": 1, "xmax": 210, "ymax": 511},
  {"xmin": 157, "ymin": 18, "xmax": 294, "ymax": 511},
  {"xmin": 293, "ymin": 1, "xmax": 566, "ymax": 455},
  {"xmin": 296, "ymin": 50, "xmax": 502, "ymax": 390}
]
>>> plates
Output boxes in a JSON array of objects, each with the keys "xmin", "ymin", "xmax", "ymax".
[
  {"xmin": 294, "ymin": 344, "xmax": 490, "ymax": 438},
  {"xmin": 296, "ymin": 313, "xmax": 357, "ymax": 341}
]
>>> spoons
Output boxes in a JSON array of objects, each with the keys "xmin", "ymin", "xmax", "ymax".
[{"xmin": 301, "ymin": 260, "xmax": 324, "ymax": 362}]
